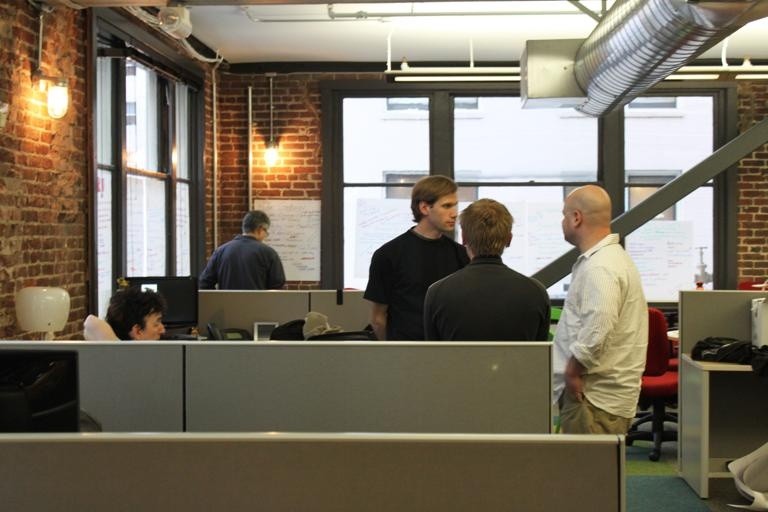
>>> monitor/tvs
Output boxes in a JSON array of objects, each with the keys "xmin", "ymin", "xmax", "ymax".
[
  {"xmin": 117, "ymin": 276, "xmax": 199, "ymax": 340},
  {"xmin": 0, "ymin": 348, "xmax": 80, "ymax": 432}
]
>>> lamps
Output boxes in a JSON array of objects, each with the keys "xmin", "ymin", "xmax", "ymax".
[
  {"xmin": 264, "ymin": 79, "xmax": 279, "ymax": 168},
  {"xmin": 32, "ymin": 0, "xmax": 70, "ymax": 120},
  {"xmin": 14, "ymin": 285, "xmax": 72, "ymax": 341}
]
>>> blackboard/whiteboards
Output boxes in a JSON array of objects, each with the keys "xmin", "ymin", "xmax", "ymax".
[{"xmin": 253, "ymin": 197, "xmax": 322, "ymax": 281}]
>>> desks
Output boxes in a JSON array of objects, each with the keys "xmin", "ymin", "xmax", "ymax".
[{"xmin": 679, "ymin": 291, "xmax": 768, "ymax": 497}]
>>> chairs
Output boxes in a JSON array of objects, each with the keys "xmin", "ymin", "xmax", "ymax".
[{"xmin": 625, "ymin": 307, "xmax": 683, "ymax": 465}]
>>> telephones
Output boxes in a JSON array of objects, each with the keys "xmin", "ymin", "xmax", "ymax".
[{"xmin": 207, "ymin": 322, "xmax": 252, "ymax": 340}]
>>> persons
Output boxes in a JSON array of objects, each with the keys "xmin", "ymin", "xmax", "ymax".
[
  {"xmin": 421, "ymin": 197, "xmax": 553, "ymax": 346},
  {"xmin": 195, "ymin": 209, "xmax": 286, "ymax": 292},
  {"xmin": 80, "ymin": 285, "xmax": 166, "ymax": 342},
  {"xmin": 545, "ymin": 183, "xmax": 651, "ymax": 440},
  {"xmin": 360, "ymin": 173, "xmax": 471, "ymax": 342}
]
